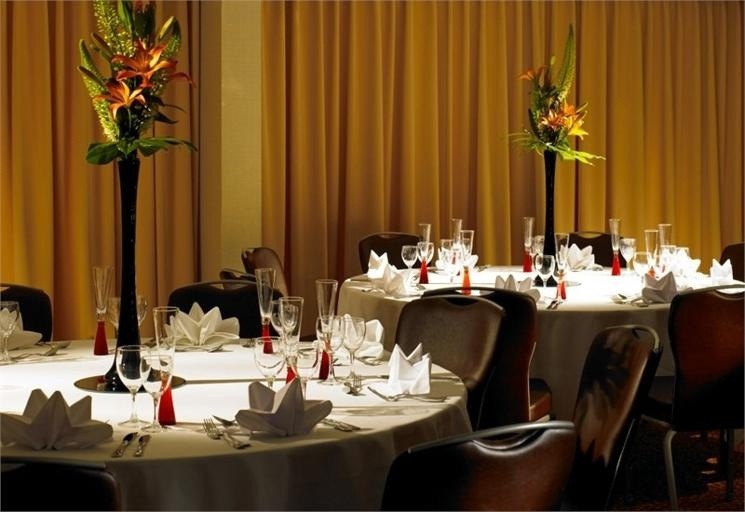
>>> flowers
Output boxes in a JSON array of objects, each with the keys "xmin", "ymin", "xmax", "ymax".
[
  {"xmin": 498, "ymin": 22, "xmax": 608, "ymax": 172},
  {"xmin": 74, "ymin": 0, "xmax": 203, "ymax": 167}
]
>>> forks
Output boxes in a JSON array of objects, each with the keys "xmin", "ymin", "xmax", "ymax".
[
  {"xmin": 367, "ymin": 386, "xmax": 444, "ymax": 403},
  {"xmin": 202, "ymin": 418, "xmax": 250, "ymax": 449}
]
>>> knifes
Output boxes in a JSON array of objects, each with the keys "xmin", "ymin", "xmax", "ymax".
[
  {"xmin": 134, "ymin": 434, "xmax": 151, "ymax": 458},
  {"xmin": 111, "ymin": 432, "xmax": 139, "ymax": 459}
]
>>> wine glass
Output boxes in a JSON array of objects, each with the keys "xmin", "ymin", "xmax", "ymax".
[
  {"xmin": 314, "ymin": 278, "xmax": 339, "ymax": 381},
  {"xmin": 316, "ymin": 317, "xmax": 346, "ymax": 385},
  {"xmin": 255, "ymin": 267, "xmax": 276, "ymax": 353},
  {"xmin": 269, "ymin": 300, "xmax": 299, "ymax": 343},
  {"xmin": 254, "ymin": 338, "xmax": 287, "ymax": 392},
  {"xmin": 92, "ymin": 265, "xmax": 115, "ymax": 354},
  {"xmin": 153, "ymin": 305, "xmax": 178, "ymax": 426},
  {"xmin": 135, "ymin": 295, "xmax": 149, "ymax": 326},
  {"xmin": 115, "ymin": 344, "xmax": 153, "ymax": 431},
  {"xmin": 139, "ymin": 354, "xmax": 173, "ymax": 433},
  {"xmin": 0, "ymin": 299, "xmax": 22, "ymax": 365},
  {"xmin": 340, "ymin": 316, "xmax": 366, "ymax": 390},
  {"xmin": 401, "ymin": 216, "xmax": 691, "ymax": 312},
  {"xmin": 107, "ymin": 297, "xmax": 122, "ymax": 331},
  {"xmin": 279, "ymin": 294, "xmax": 307, "ymax": 385}
]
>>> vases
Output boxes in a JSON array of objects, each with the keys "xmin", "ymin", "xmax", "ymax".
[
  {"xmin": 543, "ymin": 151, "xmax": 558, "ymax": 268},
  {"xmin": 104, "ymin": 160, "xmax": 139, "ymax": 391}
]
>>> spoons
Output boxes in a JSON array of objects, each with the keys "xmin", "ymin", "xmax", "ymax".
[
  {"xmin": 41, "ymin": 341, "xmax": 71, "ymax": 350},
  {"xmin": 213, "ymin": 415, "xmax": 237, "ymax": 426},
  {"xmin": 11, "ymin": 347, "xmax": 56, "ymax": 360}
]
